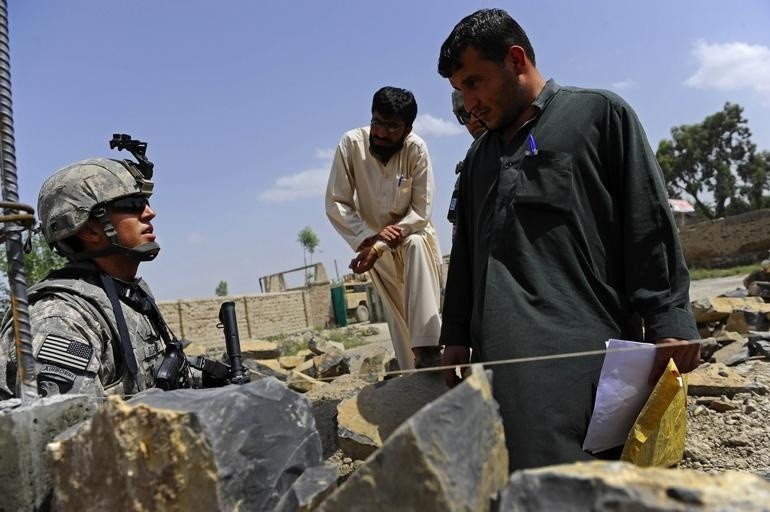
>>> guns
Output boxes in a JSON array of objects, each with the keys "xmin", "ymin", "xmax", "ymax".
[
  {"xmin": 155, "ymin": 341, "xmax": 189, "ymax": 390},
  {"xmin": 212, "ymin": 302, "xmax": 250, "ymax": 385}
]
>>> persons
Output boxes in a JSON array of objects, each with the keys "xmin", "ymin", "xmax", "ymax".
[
  {"xmin": 325, "ymin": 87, "xmax": 441, "ymax": 376},
  {"xmin": 438, "ymin": 8, "xmax": 702, "ymax": 470},
  {"xmin": 0, "ymin": 157, "xmax": 287, "ymax": 402},
  {"xmin": 448, "ymin": 91, "xmax": 487, "ymax": 223}
]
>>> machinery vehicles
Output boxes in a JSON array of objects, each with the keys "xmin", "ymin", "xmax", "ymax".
[{"xmin": 341, "ymin": 274, "xmax": 369, "ymax": 321}]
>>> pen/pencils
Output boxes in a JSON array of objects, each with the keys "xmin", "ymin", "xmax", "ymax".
[
  {"xmin": 398, "ymin": 174, "xmax": 404, "ymax": 186},
  {"xmin": 529, "ymin": 134, "xmax": 538, "ymax": 161}
]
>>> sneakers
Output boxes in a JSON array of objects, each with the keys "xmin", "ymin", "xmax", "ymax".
[{"xmin": 413, "ymin": 347, "xmax": 444, "ymax": 372}]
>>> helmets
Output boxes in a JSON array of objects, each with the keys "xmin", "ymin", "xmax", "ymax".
[
  {"xmin": 37, "ymin": 157, "xmax": 153, "ymax": 244},
  {"xmin": 451, "ymin": 90, "xmax": 464, "ymax": 114}
]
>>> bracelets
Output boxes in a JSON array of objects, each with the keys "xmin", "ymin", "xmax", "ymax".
[{"xmin": 371, "ymin": 243, "xmax": 384, "ymax": 259}]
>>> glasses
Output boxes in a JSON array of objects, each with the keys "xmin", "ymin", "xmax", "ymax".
[
  {"xmin": 371, "ymin": 119, "xmax": 401, "ymax": 132},
  {"xmin": 108, "ymin": 196, "xmax": 150, "ymax": 213},
  {"xmin": 456, "ymin": 111, "xmax": 470, "ymax": 125}
]
